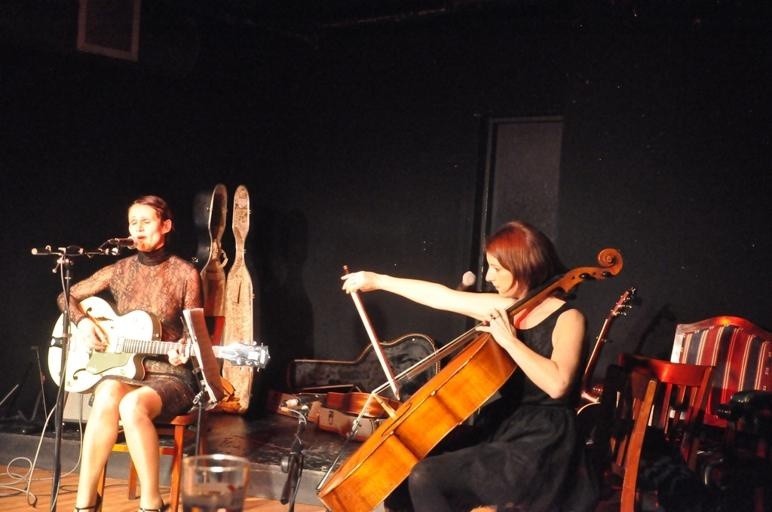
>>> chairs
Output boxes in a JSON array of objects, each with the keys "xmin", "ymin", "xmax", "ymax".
[
  {"xmin": 618, "ymin": 353, "xmax": 719, "ymax": 475},
  {"xmin": 653, "ymin": 315, "xmax": 771, "ymax": 510},
  {"xmin": 92, "ymin": 316, "xmax": 224, "ymax": 512},
  {"xmin": 597, "ymin": 365, "xmax": 658, "ymax": 512}
]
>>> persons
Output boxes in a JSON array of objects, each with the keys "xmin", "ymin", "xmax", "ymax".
[
  {"xmin": 341, "ymin": 220, "xmax": 600, "ymax": 512},
  {"xmin": 56, "ymin": 195, "xmax": 201, "ymax": 512}
]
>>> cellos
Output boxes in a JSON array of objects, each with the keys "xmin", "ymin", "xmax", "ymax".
[{"xmin": 317, "ymin": 249, "xmax": 622, "ymax": 512}]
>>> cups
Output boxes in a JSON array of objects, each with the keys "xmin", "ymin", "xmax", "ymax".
[{"xmin": 178, "ymin": 454, "xmax": 250, "ymax": 512}]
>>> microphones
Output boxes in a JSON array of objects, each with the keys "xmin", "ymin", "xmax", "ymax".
[{"xmin": 105, "ymin": 237, "xmax": 138, "ymax": 249}]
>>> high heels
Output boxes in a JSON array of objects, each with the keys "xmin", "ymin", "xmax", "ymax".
[
  {"xmin": 73, "ymin": 491, "xmax": 101, "ymax": 512},
  {"xmin": 137, "ymin": 499, "xmax": 165, "ymax": 512}
]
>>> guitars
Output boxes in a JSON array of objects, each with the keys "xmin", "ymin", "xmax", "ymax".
[
  {"xmin": 47, "ymin": 297, "xmax": 271, "ymax": 394},
  {"xmin": 575, "ymin": 286, "xmax": 636, "ymax": 418},
  {"xmin": 294, "ymin": 391, "xmax": 402, "ymax": 418}
]
